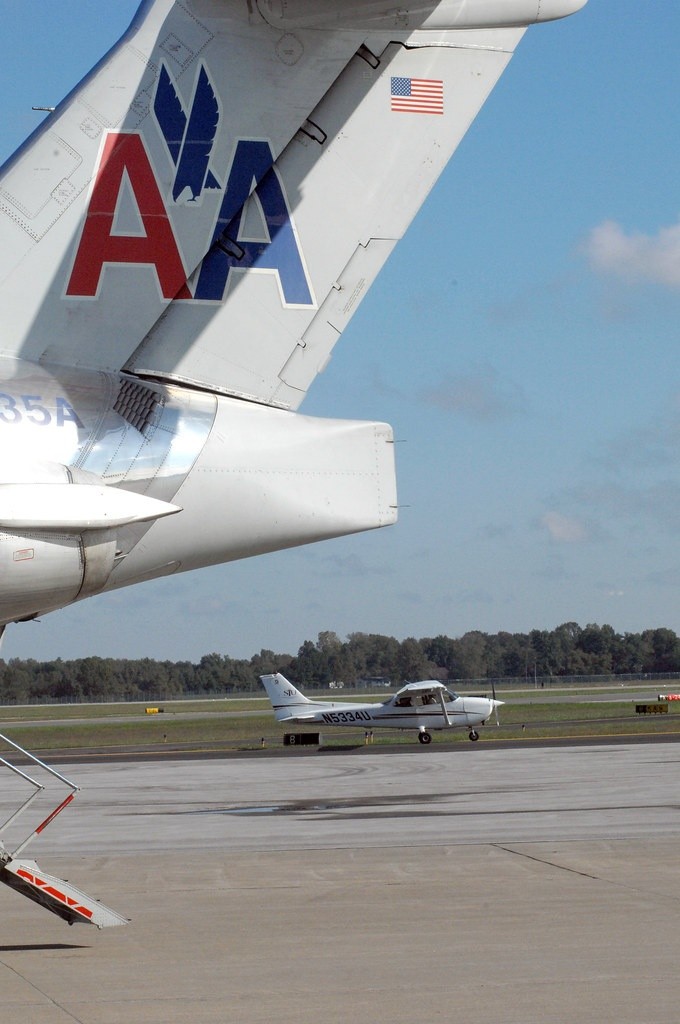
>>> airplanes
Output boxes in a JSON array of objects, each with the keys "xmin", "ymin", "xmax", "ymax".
[
  {"xmin": 1, "ymin": 0, "xmax": 590, "ymax": 928},
  {"xmin": 258, "ymin": 670, "xmax": 507, "ymax": 744}
]
